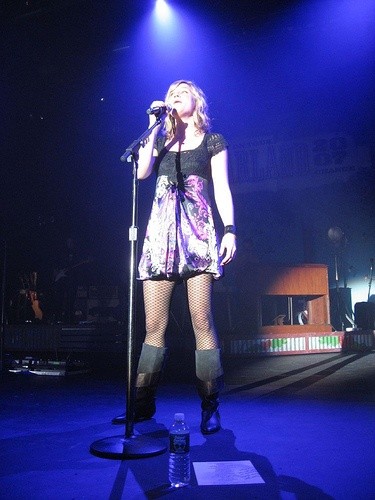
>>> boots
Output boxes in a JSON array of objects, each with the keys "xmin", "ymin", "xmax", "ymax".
[
  {"xmin": 111, "ymin": 343, "xmax": 168, "ymax": 424},
  {"xmin": 195, "ymin": 348, "xmax": 223, "ymax": 434}
]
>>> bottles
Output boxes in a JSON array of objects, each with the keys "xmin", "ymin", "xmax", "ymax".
[{"xmin": 168, "ymin": 413, "xmax": 191, "ymax": 487}]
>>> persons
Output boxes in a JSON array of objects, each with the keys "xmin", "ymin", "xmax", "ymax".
[{"xmin": 114, "ymin": 78, "xmax": 238, "ymax": 434}]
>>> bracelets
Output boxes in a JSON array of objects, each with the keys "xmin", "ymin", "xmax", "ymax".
[{"xmin": 224, "ymin": 225, "xmax": 236, "ymax": 236}]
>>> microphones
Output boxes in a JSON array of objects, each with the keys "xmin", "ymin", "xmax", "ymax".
[{"xmin": 146, "ymin": 104, "xmax": 172, "ymax": 115}]
